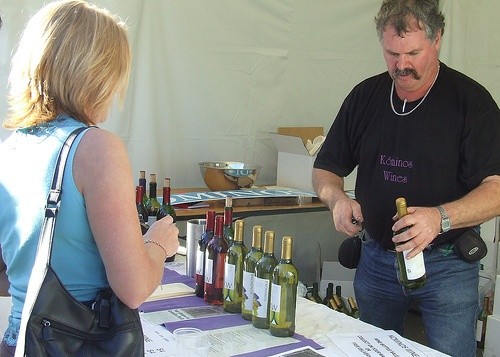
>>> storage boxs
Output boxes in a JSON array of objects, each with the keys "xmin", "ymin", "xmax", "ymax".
[
  {"xmin": 316, "ymin": 242, "xmax": 358, "ymax": 314},
  {"xmin": 269, "ymin": 127, "xmax": 326, "ymax": 194}
]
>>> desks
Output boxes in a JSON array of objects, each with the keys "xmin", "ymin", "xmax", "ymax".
[
  {"xmin": 131, "ymin": 244, "xmax": 450, "ymax": 357},
  {"xmin": 134, "ymin": 185, "xmax": 356, "ymax": 240}
]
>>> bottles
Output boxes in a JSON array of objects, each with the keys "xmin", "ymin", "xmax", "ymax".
[
  {"xmin": 144, "ymin": 174, "xmax": 161, "ymax": 228},
  {"xmin": 241, "ymin": 225, "xmax": 264, "ymax": 321},
  {"xmin": 252, "ymin": 230, "xmax": 279, "ymax": 329},
  {"xmin": 134, "ymin": 186, "xmax": 149, "ymax": 235},
  {"xmin": 155, "ymin": 178, "xmax": 176, "ymax": 262},
  {"xmin": 222, "ymin": 219, "xmax": 248, "ymax": 314},
  {"xmin": 203, "ymin": 215, "xmax": 229, "ymax": 305},
  {"xmin": 222, "ymin": 196, "xmax": 234, "ymax": 243},
  {"xmin": 268, "ymin": 235, "xmax": 298, "ymax": 337},
  {"xmin": 194, "ymin": 210, "xmax": 216, "ymax": 298},
  {"xmin": 476, "ymin": 297, "xmax": 495, "ymax": 349},
  {"xmin": 394, "ymin": 197, "xmax": 428, "ymax": 290},
  {"xmin": 138, "ymin": 171, "xmax": 150, "ymax": 206},
  {"xmin": 303, "ymin": 282, "xmax": 360, "ymax": 320}
]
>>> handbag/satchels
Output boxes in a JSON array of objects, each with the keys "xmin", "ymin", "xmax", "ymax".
[{"xmin": 14, "ymin": 125, "xmax": 145, "ymax": 357}]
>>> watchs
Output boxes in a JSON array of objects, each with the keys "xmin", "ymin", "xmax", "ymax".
[{"xmin": 436, "ymin": 207, "xmax": 450, "ymax": 234}]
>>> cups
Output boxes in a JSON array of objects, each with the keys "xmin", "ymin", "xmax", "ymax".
[{"xmin": 187, "ymin": 219, "xmax": 206, "ymax": 277}]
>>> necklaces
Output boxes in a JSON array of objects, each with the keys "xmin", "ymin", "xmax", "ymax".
[{"xmin": 390, "ymin": 65, "xmax": 441, "ymax": 115}]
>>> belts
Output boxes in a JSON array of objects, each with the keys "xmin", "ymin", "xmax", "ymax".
[{"xmin": 360, "ymin": 231, "xmax": 454, "ymax": 253}]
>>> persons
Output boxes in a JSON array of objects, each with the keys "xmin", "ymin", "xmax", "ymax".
[
  {"xmin": 0, "ymin": 0, "xmax": 180, "ymax": 357},
  {"xmin": 310, "ymin": 0, "xmax": 500, "ymax": 357}
]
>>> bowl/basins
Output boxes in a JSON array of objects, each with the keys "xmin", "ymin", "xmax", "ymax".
[{"xmin": 199, "ymin": 161, "xmax": 259, "ymax": 191}]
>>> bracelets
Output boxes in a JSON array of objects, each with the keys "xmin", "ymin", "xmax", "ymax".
[{"xmin": 144, "ymin": 240, "xmax": 168, "ymax": 257}]
